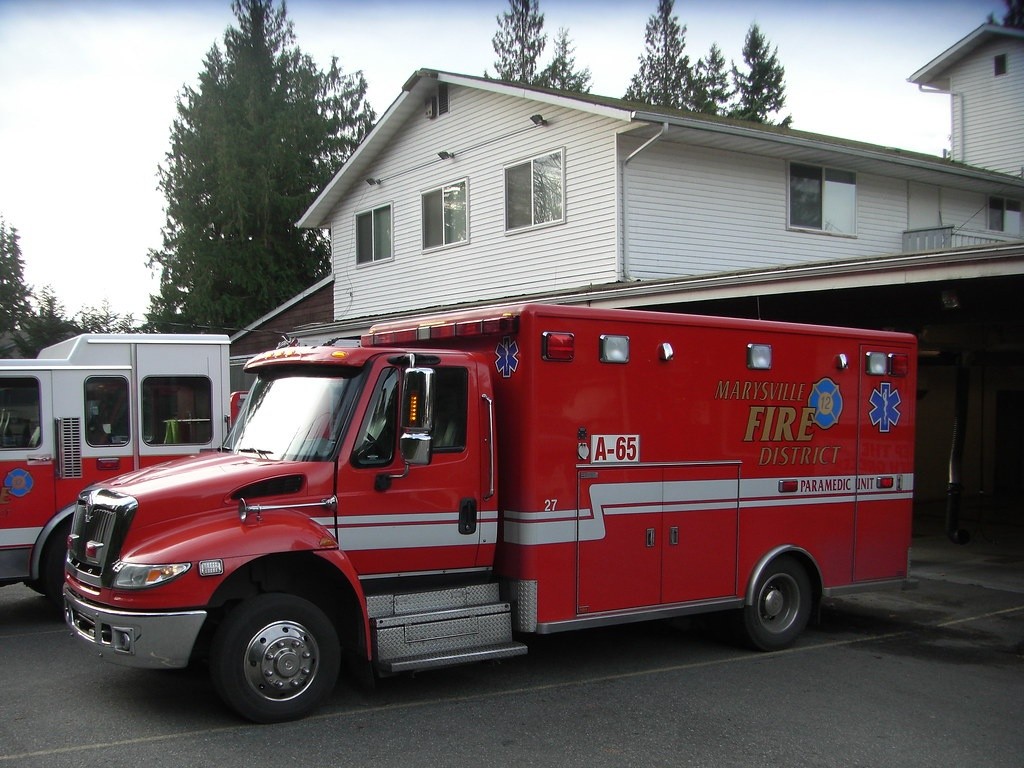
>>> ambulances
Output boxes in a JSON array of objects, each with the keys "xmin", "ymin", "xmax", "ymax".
[
  {"xmin": 0, "ymin": 333, "xmax": 234, "ymax": 597},
  {"xmin": 61, "ymin": 303, "xmax": 920, "ymax": 726}
]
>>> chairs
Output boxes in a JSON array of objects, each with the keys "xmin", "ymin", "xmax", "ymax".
[{"xmin": 27, "ymin": 426, "xmax": 41, "ymax": 448}]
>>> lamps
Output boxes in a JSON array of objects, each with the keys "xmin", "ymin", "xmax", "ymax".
[
  {"xmin": 438, "ymin": 151, "xmax": 454, "ymax": 159},
  {"xmin": 530, "ymin": 114, "xmax": 548, "ymax": 126},
  {"xmin": 365, "ymin": 178, "xmax": 381, "ymax": 185}
]
